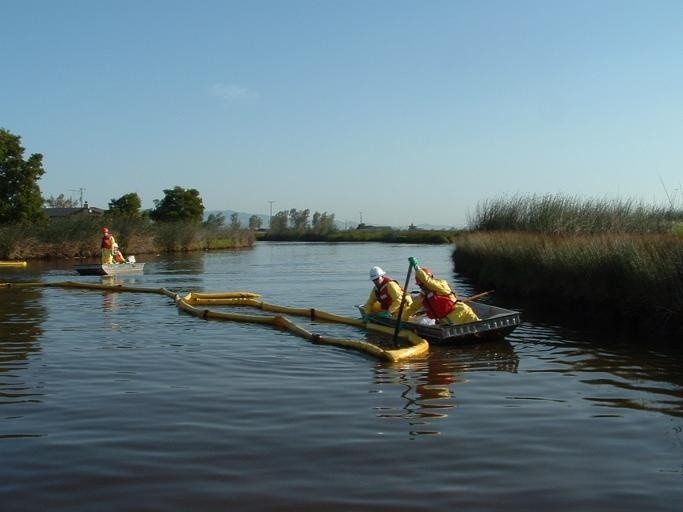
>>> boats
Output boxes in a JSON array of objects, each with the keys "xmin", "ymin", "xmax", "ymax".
[
  {"xmin": 71, "ymin": 262, "xmax": 147, "ymax": 275},
  {"xmin": 354, "ymin": 291, "xmax": 522, "ymax": 345}
]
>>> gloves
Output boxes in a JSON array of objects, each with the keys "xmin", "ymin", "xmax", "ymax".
[
  {"xmin": 377, "ymin": 311, "xmax": 390, "ymax": 318},
  {"xmin": 408, "ymin": 256, "xmax": 418, "ymax": 267}
]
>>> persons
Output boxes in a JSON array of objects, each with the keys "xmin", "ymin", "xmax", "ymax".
[
  {"xmin": 95, "ymin": 226, "xmax": 114, "ymax": 264},
  {"xmin": 112, "ymin": 242, "xmax": 126, "ymax": 265},
  {"xmin": 401, "ymin": 257, "xmax": 480, "ymax": 325},
  {"xmin": 363, "ymin": 266, "xmax": 413, "ymax": 322}
]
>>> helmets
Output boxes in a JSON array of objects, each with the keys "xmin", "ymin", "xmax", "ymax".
[
  {"xmin": 416, "ymin": 268, "xmax": 432, "ymax": 284},
  {"xmin": 114, "ymin": 243, "xmax": 118, "ymax": 248},
  {"xmin": 103, "ymin": 228, "xmax": 109, "ymax": 233},
  {"xmin": 370, "ymin": 266, "xmax": 386, "ymax": 281}
]
